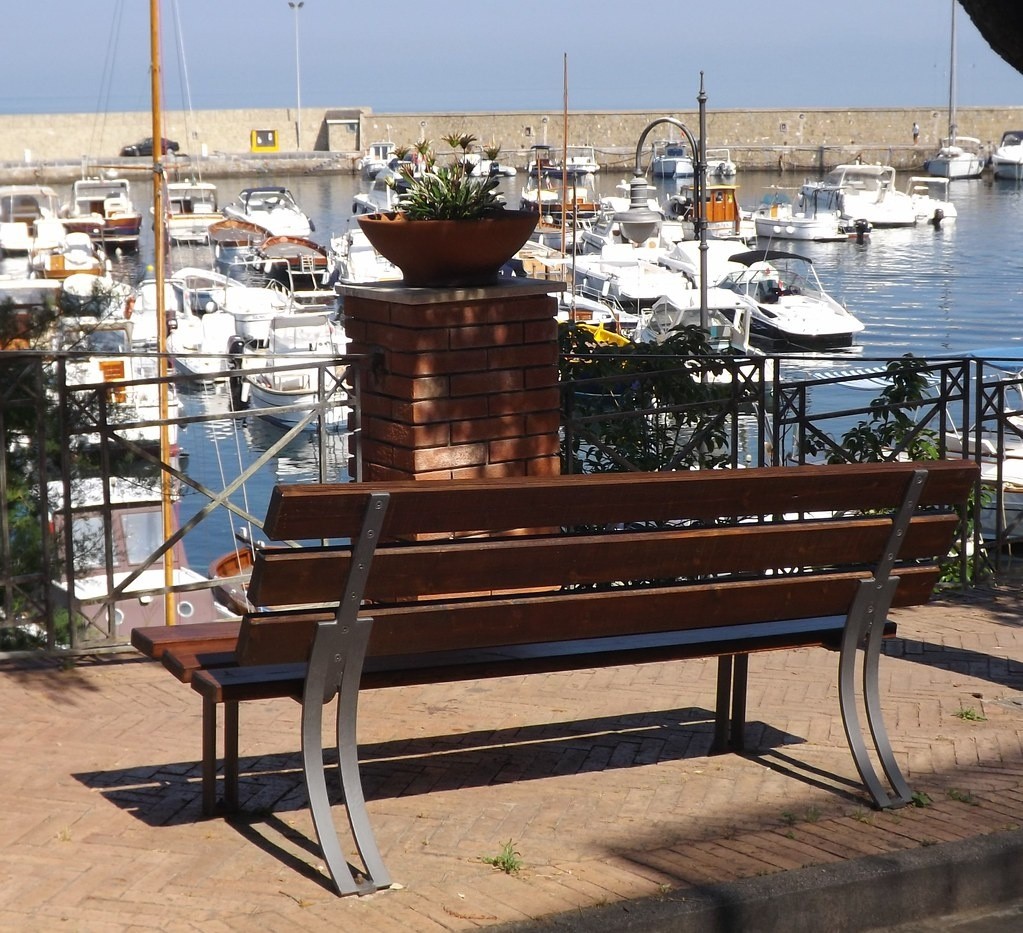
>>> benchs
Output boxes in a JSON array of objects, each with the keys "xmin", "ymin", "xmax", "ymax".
[{"xmin": 131, "ymin": 459, "xmax": 983, "ymax": 897}]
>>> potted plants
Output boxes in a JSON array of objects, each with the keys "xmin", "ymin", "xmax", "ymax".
[{"xmin": 356, "ymin": 130, "xmax": 541, "ymax": 288}]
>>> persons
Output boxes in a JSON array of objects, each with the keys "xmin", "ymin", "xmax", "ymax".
[
  {"xmin": 0, "ymin": 331, "xmax": 31, "ymax": 352},
  {"xmin": 911, "ymin": 122, "xmax": 920, "ymax": 146}
]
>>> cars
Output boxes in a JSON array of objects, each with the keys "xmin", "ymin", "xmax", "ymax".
[{"xmin": 120, "ymin": 134, "xmax": 180, "ymax": 157}]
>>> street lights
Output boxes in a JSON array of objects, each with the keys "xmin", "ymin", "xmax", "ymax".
[{"xmin": 287, "ymin": 1, "xmax": 304, "ymax": 152}]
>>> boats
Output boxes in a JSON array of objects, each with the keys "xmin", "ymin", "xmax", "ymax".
[
  {"xmin": 987, "ymin": 131, "xmax": 1022, "ymax": 182},
  {"xmin": 716, "ymin": 226, "xmax": 864, "ymax": 353},
  {"xmin": 2, "ymin": 101, "xmax": 779, "ymax": 658},
  {"xmin": 806, "ymin": 163, "xmax": 921, "ymax": 229},
  {"xmin": 742, "ymin": 180, "xmax": 876, "ymax": 243},
  {"xmin": 924, "ymin": 1, "xmax": 987, "ymax": 177},
  {"xmin": 817, "ymin": 368, "xmax": 1023, "ymax": 544},
  {"xmin": 903, "ymin": 175, "xmax": 958, "ymax": 226}
]
